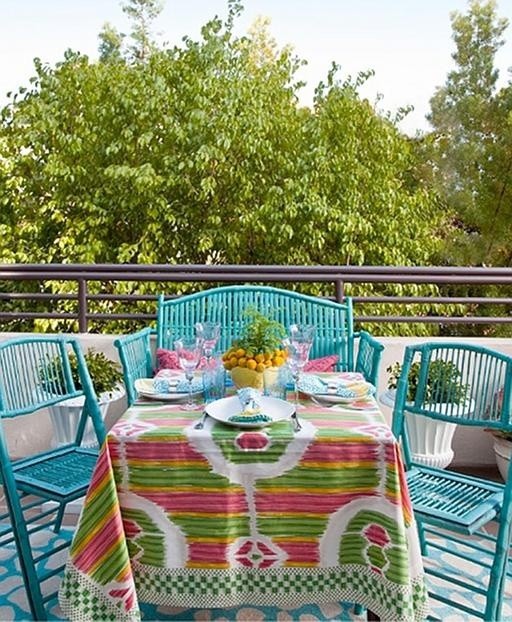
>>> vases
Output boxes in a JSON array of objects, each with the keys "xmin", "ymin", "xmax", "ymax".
[{"xmin": 492, "ymin": 434, "xmax": 511, "ymax": 484}]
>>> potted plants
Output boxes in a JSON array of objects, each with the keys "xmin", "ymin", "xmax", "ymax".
[
  {"xmin": 30, "ymin": 346, "xmax": 126, "ymax": 516},
  {"xmin": 379, "ymin": 358, "xmax": 475, "ymax": 470}
]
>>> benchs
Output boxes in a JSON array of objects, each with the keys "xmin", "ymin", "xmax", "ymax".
[{"xmin": 113, "ymin": 286, "xmax": 386, "ymax": 405}]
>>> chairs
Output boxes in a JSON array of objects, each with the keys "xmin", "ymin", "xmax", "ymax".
[
  {"xmin": 353, "ymin": 340, "xmax": 512, "ymax": 622},
  {"xmin": 0, "ymin": 333, "xmax": 108, "ymax": 622}
]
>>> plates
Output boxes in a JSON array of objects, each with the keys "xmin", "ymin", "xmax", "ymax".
[
  {"xmin": 296, "ymin": 379, "xmax": 377, "ymax": 404},
  {"xmin": 134, "ymin": 378, "xmax": 203, "ymax": 402},
  {"xmin": 205, "ymin": 396, "xmax": 295, "ymax": 428}
]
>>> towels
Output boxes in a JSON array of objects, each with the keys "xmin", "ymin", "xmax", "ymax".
[
  {"xmin": 227, "ymin": 386, "xmax": 273, "ymax": 424},
  {"xmin": 134, "ymin": 374, "xmax": 203, "ymax": 395},
  {"xmin": 293, "ymin": 371, "xmax": 377, "ymax": 397}
]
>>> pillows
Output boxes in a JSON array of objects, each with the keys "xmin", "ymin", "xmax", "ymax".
[{"xmin": 152, "ymin": 347, "xmax": 338, "ymax": 373}]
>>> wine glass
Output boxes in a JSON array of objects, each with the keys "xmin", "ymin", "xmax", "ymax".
[
  {"xmin": 174, "ymin": 322, "xmax": 220, "ymax": 412},
  {"xmin": 281, "ymin": 324, "xmax": 315, "ymax": 403}
]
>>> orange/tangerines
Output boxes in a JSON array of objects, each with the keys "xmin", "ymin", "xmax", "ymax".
[{"xmin": 222, "ymin": 346, "xmax": 289, "ymax": 373}]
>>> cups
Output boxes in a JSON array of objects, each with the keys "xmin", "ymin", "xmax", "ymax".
[
  {"xmin": 202, "ymin": 366, "xmax": 226, "ymax": 403},
  {"xmin": 263, "ymin": 368, "xmax": 287, "ymax": 402}
]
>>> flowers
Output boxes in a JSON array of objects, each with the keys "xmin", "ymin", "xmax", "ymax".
[{"xmin": 481, "ymin": 385, "xmax": 512, "ymax": 438}]
